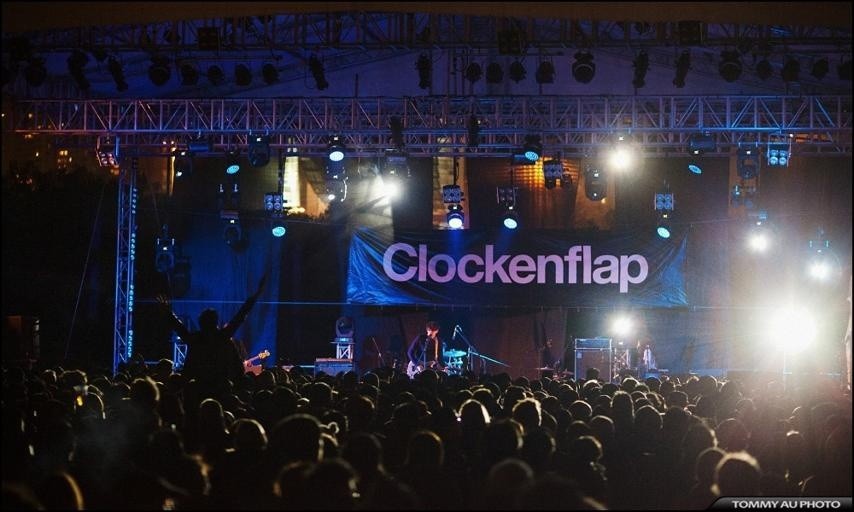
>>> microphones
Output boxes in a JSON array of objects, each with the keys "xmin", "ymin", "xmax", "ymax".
[
  {"xmin": 452, "ymin": 328, "xmax": 457, "ymax": 341},
  {"xmin": 426, "ymin": 331, "xmax": 433, "ymax": 342}
]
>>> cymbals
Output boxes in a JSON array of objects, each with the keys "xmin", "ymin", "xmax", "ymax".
[{"xmin": 444, "ymin": 351, "xmax": 466, "ymax": 357}]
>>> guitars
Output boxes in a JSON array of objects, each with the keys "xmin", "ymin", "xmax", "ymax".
[
  {"xmin": 407, "ymin": 360, "xmax": 462, "ymax": 380},
  {"xmin": 243, "ymin": 350, "xmax": 270, "ymax": 365}
]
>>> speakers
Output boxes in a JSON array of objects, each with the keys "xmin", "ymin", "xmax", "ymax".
[{"xmin": 574, "ymin": 349, "xmax": 612, "ymax": 383}]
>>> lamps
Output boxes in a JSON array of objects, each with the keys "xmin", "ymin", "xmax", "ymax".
[
  {"xmin": 1, "ymin": 25, "xmax": 854, "ymax": 92},
  {"xmin": 49, "ymin": 134, "xmax": 794, "ymax": 271}
]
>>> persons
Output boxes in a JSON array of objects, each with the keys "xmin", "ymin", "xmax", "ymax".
[
  {"xmin": 405, "ymin": 319, "xmax": 448, "ymax": 374},
  {"xmin": 540, "ymin": 336, "xmax": 561, "ymax": 377},
  {"xmin": 611, "ymin": 333, "xmax": 654, "ymax": 377},
  {"xmin": 155, "ymin": 273, "xmax": 267, "ymax": 379},
  {"xmin": 0, "ymin": 357, "xmax": 854, "ymax": 510}
]
